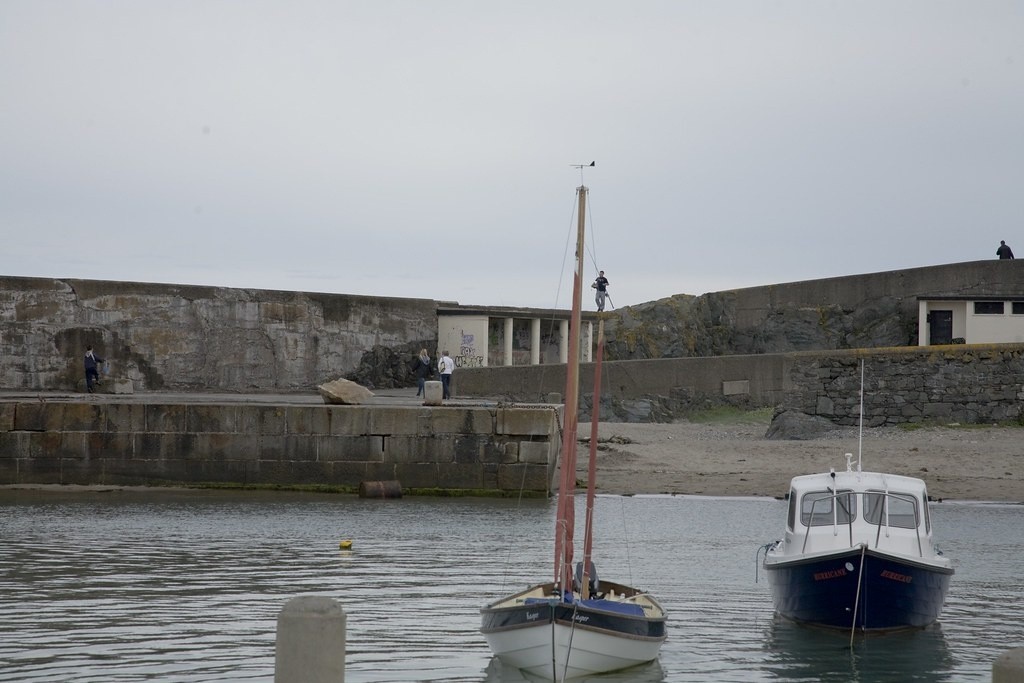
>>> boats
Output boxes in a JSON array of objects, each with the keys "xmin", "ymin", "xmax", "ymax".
[{"xmin": 754, "ymin": 357, "xmax": 957, "ymax": 637}]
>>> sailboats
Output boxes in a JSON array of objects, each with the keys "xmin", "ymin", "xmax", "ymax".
[{"xmin": 477, "ymin": 185, "xmax": 666, "ymax": 683}]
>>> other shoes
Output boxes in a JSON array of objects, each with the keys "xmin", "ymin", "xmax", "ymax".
[
  {"xmin": 95, "ymin": 382, "xmax": 101, "ymax": 386},
  {"xmin": 88, "ymin": 388, "xmax": 92, "ymax": 393},
  {"xmin": 443, "ymin": 397, "xmax": 446, "ymax": 399},
  {"xmin": 416, "ymin": 395, "xmax": 420, "ymax": 397}
]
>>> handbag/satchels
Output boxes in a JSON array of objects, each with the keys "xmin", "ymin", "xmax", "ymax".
[{"xmin": 440, "ymin": 357, "xmax": 445, "ymax": 372}]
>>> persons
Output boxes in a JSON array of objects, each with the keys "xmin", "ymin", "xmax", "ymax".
[
  {"xmin": 84, "ymin": 345, "xmax": 106, "ymax": 393},
  {"xmin": 437, "ymin": 351, "xmax": 454, "ymax": 400},
  {"xmin": 996, "ymin": 240, "xmax": 1014, "ymax": 259},
  {"xmin": 412, "ymin": 349, "xmax": 430, "ymax": 399},
  {"xmin": 591, "ymin": 271, "xmax": 609, "ymax": 312}
]
